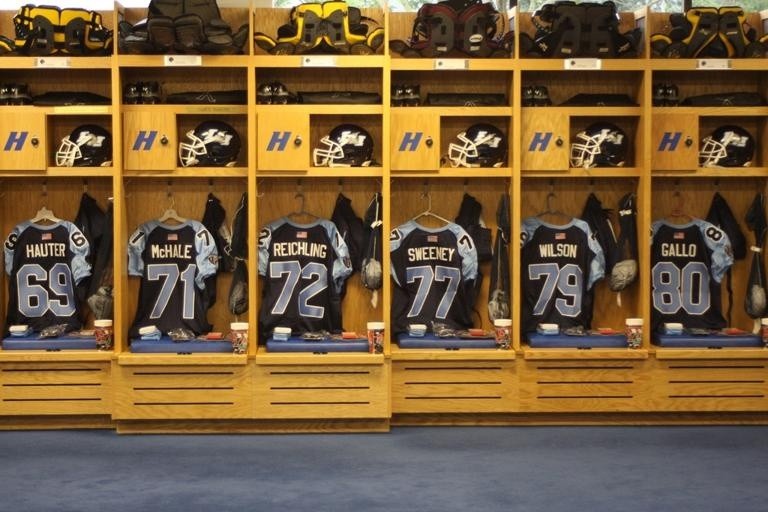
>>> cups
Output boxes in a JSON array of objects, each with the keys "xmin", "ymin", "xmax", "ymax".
[
  {"xmin": 493, "ymin": 318, "xmax": 513, "ymax": 350},
  {"xmin": 93, "ymin": 319, "xmax": 113, "ymax": 351},
  {"xmin": 625, "ymin": 317, "xmax": 643, "ymax": 349},
  {"xmin": 230, "ymin": 322, "xmax": 249, "ymax": 354},
  {"xmin": 365, "ymin": 321, "xmax": 385, "ymax": 354}
]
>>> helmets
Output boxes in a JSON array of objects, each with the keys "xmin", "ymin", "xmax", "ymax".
[
  {"xmin": 178, "ymin": 120, "xmax": 241, "ymax": 167},
  {"xmin": 447, "ymin": 123, "xmax": 507, "ymax": 168},
  {"xmin": 697, "ymin": 125, "xmax": 755, "ymax": 168},
  {"xmin": 313, "ymin": 123, "xmax": 374, "ymax": 168},
  {"xmin": 55, "ymin": 124, "xmax": 112, "ymax": 167},
  {"xmin": 569, "ymin": 121, "xmax": 628, "ymax": 171}
]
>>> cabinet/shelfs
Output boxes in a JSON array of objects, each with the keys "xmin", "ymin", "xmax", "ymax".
[{"xmin": 2, "ymin": 1, "xmax": 768, "ymax": 433}]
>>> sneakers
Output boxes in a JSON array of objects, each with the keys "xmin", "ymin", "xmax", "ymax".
[
  {"xmin": 0, "ymin": 82, "xmax": 11, "ymax": 105},
  {"xmin": 271, "ymin": 84, "xmax": 297, "ymax": 105},
  {"xmin": 521, "ymin": 85, "xmax": 535, "ymax": 107},
  {"xmin": 256, "ymin": 83, "xmax": 273, "ymax": 105},
  {"xmin": 532, "ymin": 86, "xmax": 552, "ymax": 107},
  {"xmin": 404, "ymin": 84, "xmax": 421, "ymax": 107},
  {"xmin": 124, "ymin": 81, "xmax": 141, "ymax": 104},
  {"xmin": 391, "ymin": 84, "xmax": 405, "ymax": 107},
  {"xmin": 8, "ymin": 83, "xmax": 33, "ymax": 105},
  {"xmin": 652, "ymin": 82, "xmax": 666, "ymax": 107},
  {"xmin": 664, "ymin": 86, "xmax": 679, "ymax": 107},
  {"xmin": 141, "ymin": 80, "xmax": 161, "ymax": 103}
]
